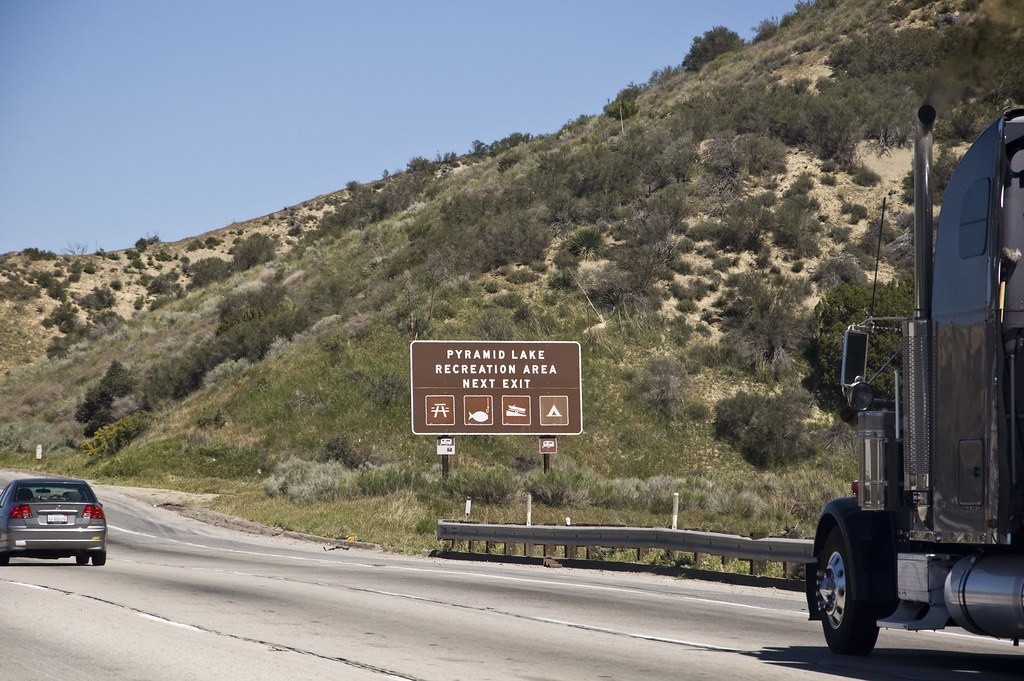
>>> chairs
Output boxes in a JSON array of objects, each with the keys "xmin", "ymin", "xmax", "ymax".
[
  {"xmin": 16, "ymin": 489, "xmax": 34, "ymax": 502},
  {"xmin": 62, "ymin": 491, "xmax": 80, "ymax": 503}
]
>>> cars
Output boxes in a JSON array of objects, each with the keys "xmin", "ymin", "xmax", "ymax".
[{"xmin": 0, "ymin": 478, "xmax": 107, "ymax": 566}]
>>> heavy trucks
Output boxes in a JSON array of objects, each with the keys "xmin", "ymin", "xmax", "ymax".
[{"xmin": 805, "ymin": 103, "xmax": 1024, "ymax": 653}]
